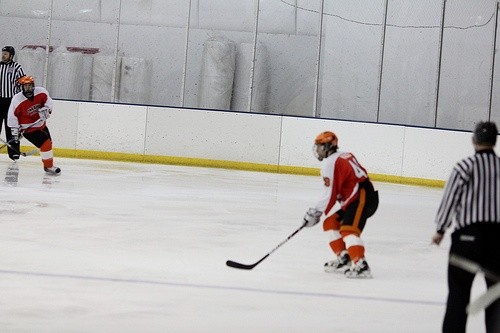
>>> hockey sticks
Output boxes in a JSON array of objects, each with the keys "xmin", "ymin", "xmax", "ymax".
[
  {"xmin": 0, "ymin": 118, "xmax": 43, "ymax": 150},
  {"xmin": 1, "ymin": 137, "xmax": 39, "ymax": 157},
  {"xmin": 464, "ymin": 280, "xmax": 500, "ymax": 316},
  {"xmin": 225, "ymin": 220, "xmax": 310, "ymax": 271}
]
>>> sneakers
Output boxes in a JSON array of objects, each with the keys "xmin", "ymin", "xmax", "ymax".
[
  {"xmin": 344, "ymin": 259, "xmax": 372, "ymax": 279},
  {"xmin": 44, "ymin": 166, "xmax": 61, "ymax": 175},
  {"xmin": 325, "ymin": 251, "xmax": 352, "ymax": 273}
]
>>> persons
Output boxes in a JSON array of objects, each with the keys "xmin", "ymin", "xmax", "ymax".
[
  {"xmin": 430, "ymin": 121, "xmax": 500, "ymax": 333},
  {"xmin": 0, "ymin": 45, "xmax": 27, "ymax": 168},
  {"xmin": 302, "ymin": 130, "xmax": 380, "ymax": 278},
  {"xmin": 6, "ymin": 75, "xmax": 62, "ymax": 174}
]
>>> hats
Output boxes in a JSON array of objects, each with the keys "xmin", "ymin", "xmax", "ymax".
[{"xmin": 475, "ymin": 122, "xmax": 500, "ymax": 138}]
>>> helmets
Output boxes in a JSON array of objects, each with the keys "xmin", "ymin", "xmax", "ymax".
[
  {"xmin": 315, "ymin": 131, "xmax": 338, "ymax": 146},
  {"xmin": 18, "ymin": 76, "xmax": 34, "ymax": 84},
  {"xmin": 2, "ymin": 46, "xmax": 15, "ymax": 55}
]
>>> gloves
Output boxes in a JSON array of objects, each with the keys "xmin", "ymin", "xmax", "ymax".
[
  {"xmin": 304, "ymin": 209, "xmax": 322, "ymax": 227},
  {"xmin": 11, "ymin": 131, "xmax": 24, "ymax": 141},
  {"xmin": 38, "ymin": 107, "xmax": 50, "ymax": 120}
]
